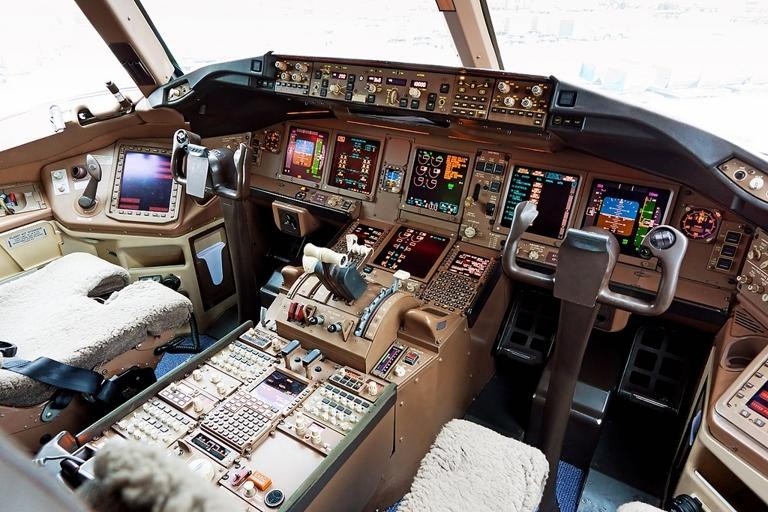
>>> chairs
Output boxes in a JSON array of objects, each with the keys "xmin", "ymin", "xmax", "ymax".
[{"xmin": 0, "ymin": 250, "xmax": 195, "ymax": 443}]
